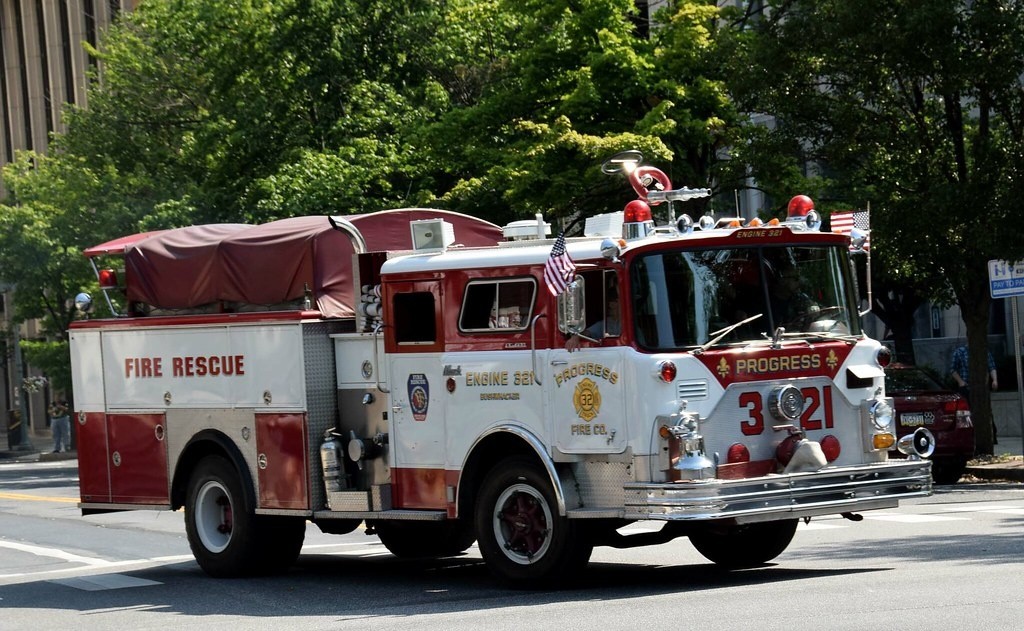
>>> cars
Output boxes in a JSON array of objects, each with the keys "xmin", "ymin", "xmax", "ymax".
[{"xmin": 884, "ymin": 362, "xmax": 973, "ymax": 485}]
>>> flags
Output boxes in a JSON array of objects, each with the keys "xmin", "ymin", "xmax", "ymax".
[{"xmin": 544, "ymin": 232, "xmax": 576, "ymax": 298}]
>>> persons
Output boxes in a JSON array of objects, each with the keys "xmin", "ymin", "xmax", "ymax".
[
  {"xmin": 950, "ymin": 341, "xmax": 998, "ymax": 458},
  {"xmin": 566, "ymin": 287, "xmax": 646, "ymax": 352},
  {"xmin": 48, "ymin": 394, "xmax": 71, "ymax": 453}
]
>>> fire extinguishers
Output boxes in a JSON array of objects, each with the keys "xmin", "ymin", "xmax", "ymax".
[{"xmin": 319, "ymin": 426, "xmax": 346, "ymax": 509}]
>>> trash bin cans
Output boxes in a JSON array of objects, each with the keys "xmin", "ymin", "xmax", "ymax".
[{"xmin": 6, "ymin": 409, "xmax": 22, "ymax": 450}]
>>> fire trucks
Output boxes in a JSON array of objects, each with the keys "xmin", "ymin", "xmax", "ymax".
[{"xmin": 66, "ymin": 151, "xmax": 934, "ymax": 589}]
